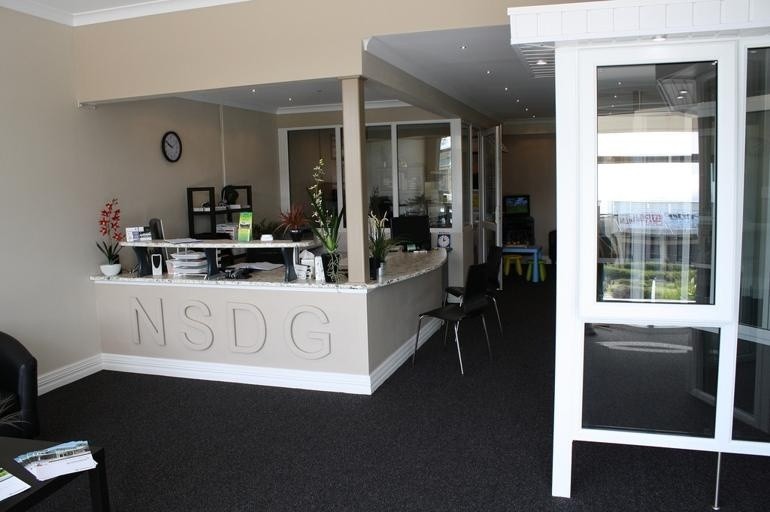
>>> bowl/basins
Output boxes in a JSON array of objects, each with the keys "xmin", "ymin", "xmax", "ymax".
[{"xmin": 100, "ymin": 263, "xmax": 122, "ymax": 276}]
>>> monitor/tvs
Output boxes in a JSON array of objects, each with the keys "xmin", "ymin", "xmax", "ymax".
[{"xmin": 503, "ymin": 195, "xmax": 530, "ymax": 215}]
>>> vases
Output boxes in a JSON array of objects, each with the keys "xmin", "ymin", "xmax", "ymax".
[
  {"xmin": 99, "ymin": 264, "xmax": 121, "ymax": 277},
  {"xmin": 321, "ymin": 253, "xmax": 340, "ymax": 283},
  {"xmin": 369, "ymin": 258, "xmax": 385, "ymax": 280}
]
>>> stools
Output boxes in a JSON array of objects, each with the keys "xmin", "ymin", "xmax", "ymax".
[
  {"xmin": 526, "ymin": 260, "xmax": 546, "ymax": 282},
  {"xmin": 504, "ymin": 254, "xmax": 523, "ymax": 276}
]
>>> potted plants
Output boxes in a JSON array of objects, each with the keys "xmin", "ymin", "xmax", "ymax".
[
  {"xmin": 259, "ymin": 218, "xmax": 277, "ymax": 242},
  {"xmin": 273, "ymin": 202, "xmax": 315, "ymax": 242}
]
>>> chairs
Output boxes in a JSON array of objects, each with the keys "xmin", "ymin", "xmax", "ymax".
[
  {"xmin": 411, "ymin": 256, "xmax": 500, "ymax": 375},
  {"xmin": 0, "ymin": 330, "xmax": 40, "ymax": 439},
  {"xmin": 442, "ymin": 245, "xmax": 503, "ymax": 342}
]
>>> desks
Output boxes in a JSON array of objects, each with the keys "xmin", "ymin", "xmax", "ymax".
[
  {"xmin": 502, "ymin": 246, "xmax": 542, "ymax": 283},
  {"xmin": 0, "ymin": 436, "xmax": 110, "ymax": 512}
]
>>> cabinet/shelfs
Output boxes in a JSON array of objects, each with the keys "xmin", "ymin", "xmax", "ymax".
[{"xmin": 187, "ymin": 185, "xmax": 253, "ymax": 258}]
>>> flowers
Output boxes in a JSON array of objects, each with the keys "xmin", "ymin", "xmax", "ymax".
[
  {"xmin": 96, "ymin": 198, "xmax": 126, "ymax": 263},
  {"xmin": 368, "ymin": 210, "xmax": 412, "ymax": 262},
  {"xmin": 306, "ymin": 158, "xmax": 346, "ymax": 287}
]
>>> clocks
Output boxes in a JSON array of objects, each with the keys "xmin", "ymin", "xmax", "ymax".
[
  {"xmin": 161, "ymin": 132, "xmax": 182, "ymax": 162},
  {"xmin": 438, "ymin": 234, "xmax": 450, "ymax": 249}
]
